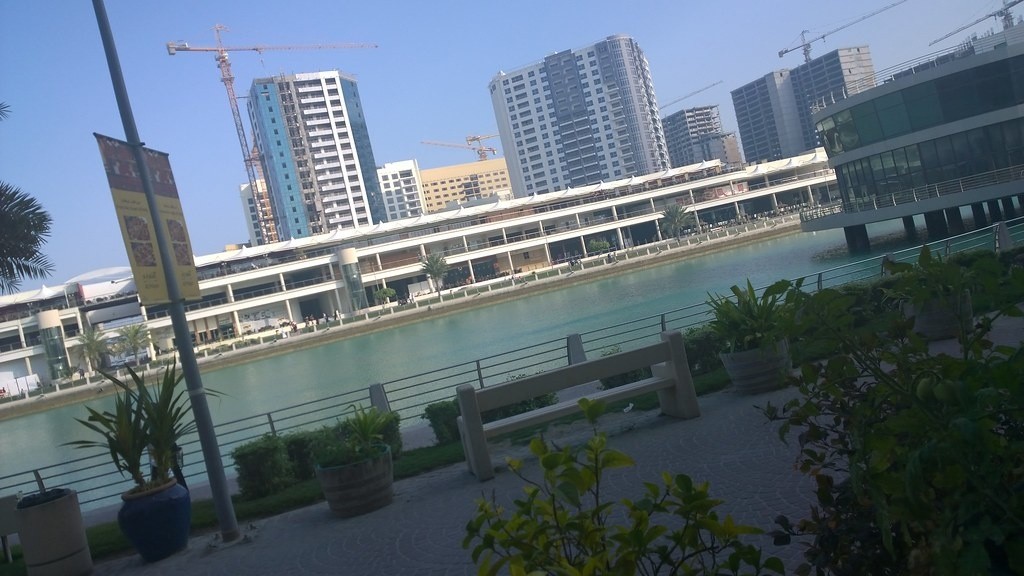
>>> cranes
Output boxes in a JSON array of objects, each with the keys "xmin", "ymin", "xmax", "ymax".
[
  {"xmin": 658, "ymin": 80, "xmax": 725, "ymax": 110},
  {"xmin": 164, "ymin": 24, "xmax": 378, "ymax": 246},
  {"xmin": 778, "ymin": 0, "xmax": 906, "ymax": 64},
  {"xmin": 928, "ymin": 0, "xmax": 1024, "ymax": 48},
  {"xmin": 419, "ymin": 133, "xmax": 502, "ymax": 162}
]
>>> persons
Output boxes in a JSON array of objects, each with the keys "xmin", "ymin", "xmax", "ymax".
[
  {"xmin": 310, "ymin": 308, "xmax": 343, "ymax": 325},
  {"xmin": 518, "ymin": 276, "xmax": 525, "ymax": 283},
  {"xmin": 3, "ymin": 387, "xmax": 8, "ymax": 398},
  {"xmin": 607, "ymin": 252, "xmax": 619, "ymax": 263},
  {"xmin": 79, "ymin": 368, "xmax": 85, "ymax": 379},
  {"xmin": 291, "ymin": 322, "xmax": 298, "ymax": 332},
  {"xmin": 569, "ymin": 258, "xmax": 579, "ymax": 266},
  {"xmin": 465, "ymin": 275, "xmax": 472, "ymax": 285},
  {"xmin": 417, "ymin": 253, "xmax": 422, "ymax": 262},
  {"xmin": 397, "ymin": 296, "xmax": 408, "ymax": 306},
  {"xmin": 305, "ymin": 316, "xmax": 310, "ymax": 328}
]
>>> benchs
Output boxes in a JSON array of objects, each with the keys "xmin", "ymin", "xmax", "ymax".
[{"xmin": 455, "ymin": 330, "xmax": 700, "ymax": 481}]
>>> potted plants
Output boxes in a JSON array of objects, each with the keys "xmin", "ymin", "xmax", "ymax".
[
  {"xmin": 704, "ymin": 274, "xmax": 793, "ymax": 394},
  {"xmin": 883, "ymin": 244, "xmax": 982, "ymax": 341},
  {"xmin": 71, "ymin": 359, "xmax": 229, "ymax": 565},
  {"xmin": 308, "ymin": 404, "xmax": 398, "ymax": 517}
]
